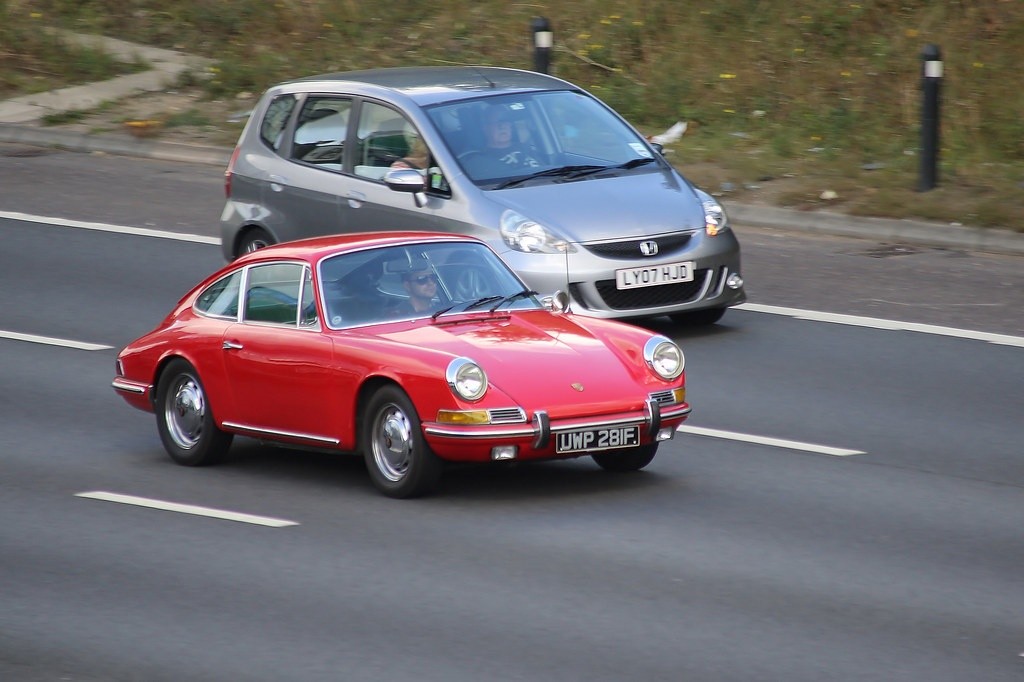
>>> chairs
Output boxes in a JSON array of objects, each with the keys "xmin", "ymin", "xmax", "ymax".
[
  {"xmin": 368, "ymin": 118, "xmax": 409, "ymax": 169},
  {"xmin": 467, "ymin": 104, "xmax": 521, "ymax": 151},
  {"xmin": 338, "ymin": 263, "xmax": 387, "ymax": 321}
]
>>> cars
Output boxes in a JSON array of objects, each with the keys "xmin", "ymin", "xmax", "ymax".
[{"xmin": 218, "ymin": 63, "xmax": 747, "ymax": 332}]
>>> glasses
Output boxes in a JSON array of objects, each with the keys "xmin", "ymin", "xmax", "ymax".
[{"xmin": 410, "ymin": 273, "xmax": 436, "ymax": 285}]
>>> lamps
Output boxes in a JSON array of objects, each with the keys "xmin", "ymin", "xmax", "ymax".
[
  {"xmin": 531, "ymin": 17, "xmax": 554, "ymax": 59},
  {"xmin": 921, "ymin": 43, "xmax": 944, "ymax": 96}
]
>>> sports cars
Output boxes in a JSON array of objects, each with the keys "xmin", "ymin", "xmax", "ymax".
[{"xmin": 110, "ymin": 230, "xmax": 692, "ymax": 500}]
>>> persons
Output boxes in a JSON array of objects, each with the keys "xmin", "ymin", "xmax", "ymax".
[
  {"xmin": 392, "ymin": 121, "xmax": 430, "ymax": 189},
  {"xmin": 387, "ymin": 260, "xmax": 454, "ymax": 319},
  {"xmin": 470, "ymin": 104, "xmax": 546, "ymax": 179}
]
